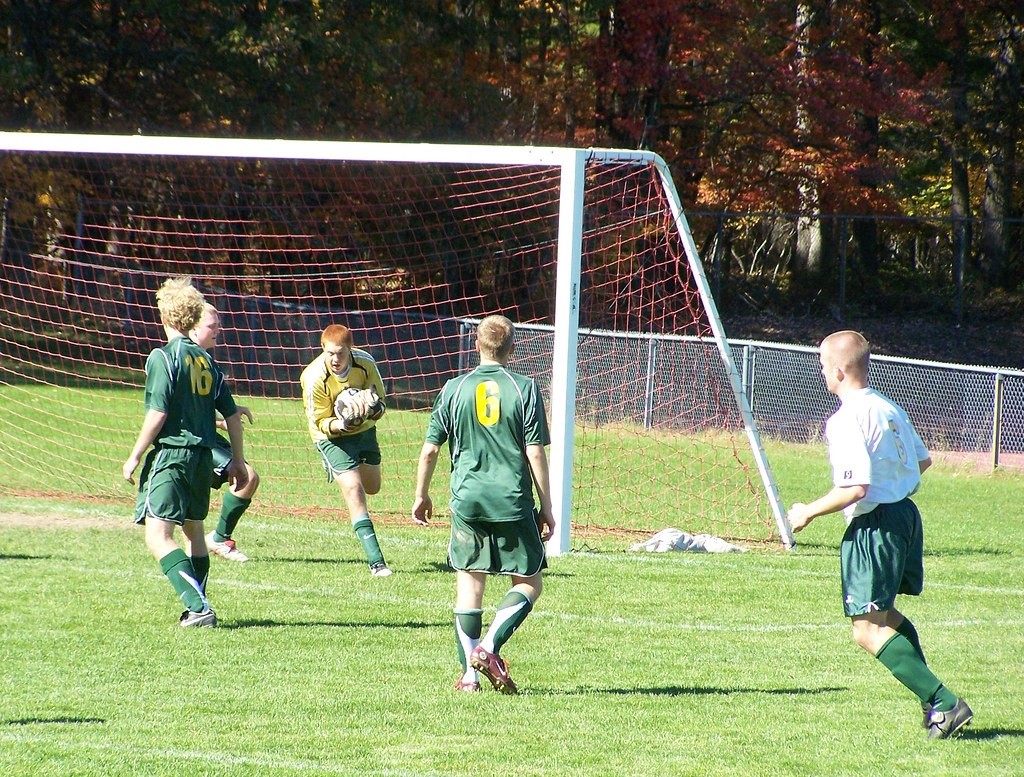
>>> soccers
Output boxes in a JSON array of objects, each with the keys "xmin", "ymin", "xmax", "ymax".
[{"xmin": 332, "ymin": 387, "xmax": 367, "ymax": 422}]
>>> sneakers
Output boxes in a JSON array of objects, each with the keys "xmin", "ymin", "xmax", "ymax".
[
  {"xmin": 923, "ymin": 697, "xmax": 973, "ymax": 742},
  {"xmin": 204, "ymin": 530, "xmax": 248, "ymax": 562},
  {"xmin": 179, "ymin": 608, "xmax": 216, "ymax": 629},
  {"xmin": 369, "ymin": 563, "xmax": 392, "ymax": 577},
  {"xmin": 470, "ymin": 644, "xmax": 518, "ymax": 695},
  {"xmin": 455, "ymin": 673, "xmax": 481, "ymax": 694}
]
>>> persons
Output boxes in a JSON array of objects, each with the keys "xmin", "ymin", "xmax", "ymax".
[
  {"xmin": 299, "ymin": 324, "xmax": 394, "ymax": 576},
  {"xmin": 411, "ymin": 315, "xmax": 556, "ymax": 694},
  {"xmin": 786, "ymin": 330, "xmax": 973, "ymax": 740},
  {"xmin": 188, "ymin": 302, "xmax": 260, "ymax": 564},
  {"xmin": 122, "ymin": 275, "xmax": 249, "ymax": 628}
]
